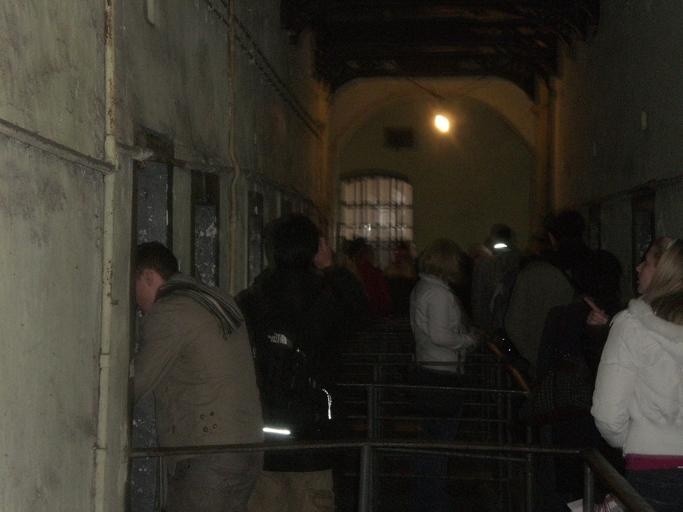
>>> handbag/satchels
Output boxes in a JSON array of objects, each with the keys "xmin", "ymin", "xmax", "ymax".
[{"xmin": 413, "ymin": 365, "xmax": 468, "ymax": 417}]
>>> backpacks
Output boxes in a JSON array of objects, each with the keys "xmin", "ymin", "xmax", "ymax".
[{"xmin": 238, "ymin": 283, "xmax": 324, "ymax": 431}]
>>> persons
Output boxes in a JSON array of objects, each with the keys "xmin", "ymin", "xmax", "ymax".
[
  {"xmin": 338, "ymin": 205, "xmax": 628, "ymax": 512},
  {"xmin": 132, "ymin": 238, "xmax": 265, "ymax": 512},
  {"xmin": 231, "ymin": 211, "xmax": 374, "ymax": 511},
  {"xmin": 589, "ymin": 234, "xmax": 682, "ymax": 511}
]
